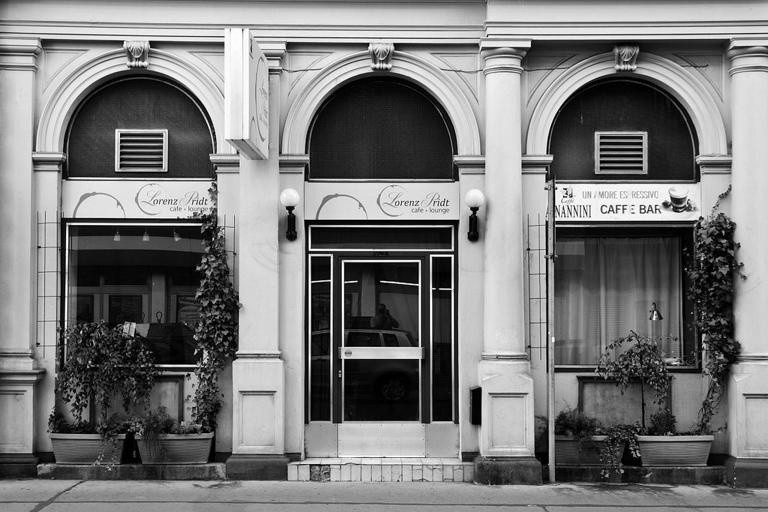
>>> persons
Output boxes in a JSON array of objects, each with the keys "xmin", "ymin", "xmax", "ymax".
[{"xmin": 369, "ymin": 304, "xmax": 400, "ymax": 347}]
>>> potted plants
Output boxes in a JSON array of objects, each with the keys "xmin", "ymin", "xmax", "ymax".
[
  {"xmin": 46, "ymin": 405, "xmax": 134, "ymax": 475},
  {"xmin": 632, "ymin": 407, "xmax": 728, "ymax": 466},
  {"xmin": 589, "ymin": 421, "xmax": 642, "ymax": 483},
  {"xmin": 141, "ymin": 404, "xmax": 216, "ymax": 465},
  {"xmin": 534, "ymin": 396, "xmax": 601, "ymax": 465}
]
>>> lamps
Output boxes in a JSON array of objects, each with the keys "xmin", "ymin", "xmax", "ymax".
[
  {"xmin": 464, "ymin": 188, "xmax": 485, "ymax": 241},
  {"xmin": 280, "ymin": 187, "xmax": 300, "ymax": 242},
  {"xmin": 112, "ymin": 229, "xmax": 183, "ymax": 243}
]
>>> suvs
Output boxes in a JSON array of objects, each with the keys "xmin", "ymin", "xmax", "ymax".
[{"xmin": 311, "ymin": 329, "xmax": 415, "ymax": 400}]
